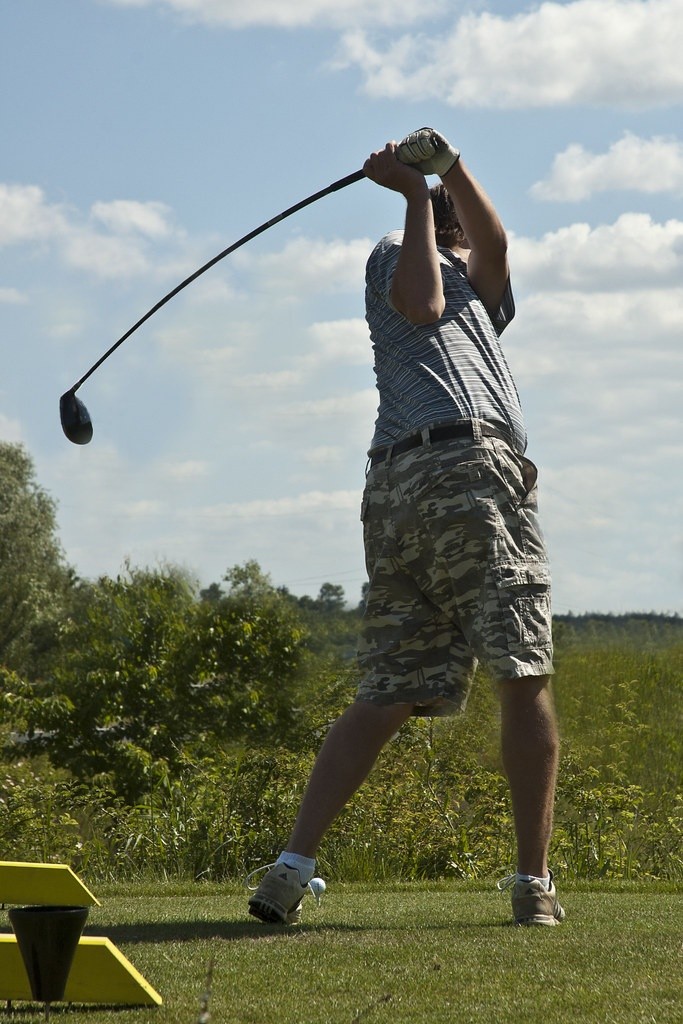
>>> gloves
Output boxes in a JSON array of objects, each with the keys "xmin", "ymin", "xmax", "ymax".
[{"xmin": 394, "ymin": 127, "xmax": 461, "ymax": 178}]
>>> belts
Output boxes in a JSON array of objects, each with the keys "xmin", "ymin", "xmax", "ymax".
[{"xmin": 370, "ymin": 423, "xmax": 516, "ymax": 468}]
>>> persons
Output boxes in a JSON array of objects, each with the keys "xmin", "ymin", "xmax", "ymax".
[{"xmin": 247, "ymin": 127, "xmax": 566, "ymax": 928}]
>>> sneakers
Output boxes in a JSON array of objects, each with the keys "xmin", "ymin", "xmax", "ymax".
[
  {"xmin": 245, "ymin": 862, "xmax": 316, "ymax": 926},
  {"xmin": 496, "ymin": 870, "xmax": 567, "ymax": 928}
]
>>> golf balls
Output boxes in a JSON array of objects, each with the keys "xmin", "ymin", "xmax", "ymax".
[{"xmin": 310, "ymin": 877, "xmax": 326, "ymax": 895}]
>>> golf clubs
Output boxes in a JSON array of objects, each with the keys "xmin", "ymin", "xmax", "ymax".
[{"xmin": 58, "ymin": 136, "xmax": 442, "ymax": 445}]
